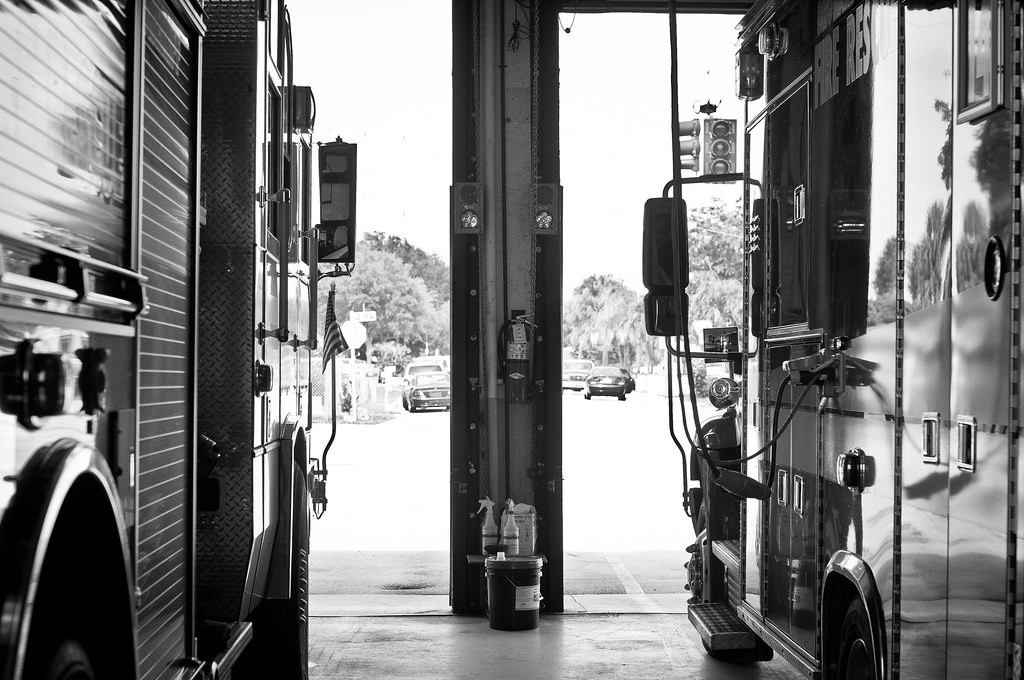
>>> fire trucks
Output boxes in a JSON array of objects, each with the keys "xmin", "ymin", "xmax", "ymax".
[
  {"xmin": 643, "ymin": 0, "xmax": 1024, "ymax": 680},
  {"xmin": 0, "ymin": 0, "xmax": 357, "ymax": 680}
]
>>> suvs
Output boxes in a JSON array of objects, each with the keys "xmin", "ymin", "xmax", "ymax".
[
  {"xmin": 563, "ymin": 359, "xmax": 596, "ymax": 391},
  {"xmin": 402, "ymin": 357, "xmax": 450, "ymax": 378}
]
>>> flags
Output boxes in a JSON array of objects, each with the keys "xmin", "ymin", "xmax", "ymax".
[{"xmin": 321, "ymin": 291, "xmax": 350, "ymax": 374}]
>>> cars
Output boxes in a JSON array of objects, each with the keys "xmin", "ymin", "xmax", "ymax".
[
  {"xmin": 402, "ymin": 371, "xmax": 450, "ymax": 413},
  {"xmin": 586, "ymin": 366, "xmax": 637, "ymax": 400}
]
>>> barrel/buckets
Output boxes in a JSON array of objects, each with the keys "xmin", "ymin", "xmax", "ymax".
[{"xmin": 485, "ymin": 555, "xmax": 542, "ymax": 631}]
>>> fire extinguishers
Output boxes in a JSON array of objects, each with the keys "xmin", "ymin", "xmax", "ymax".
[{"xmin": 501, "ymin": 313, "xmax": 538, "ymax": 404}]
